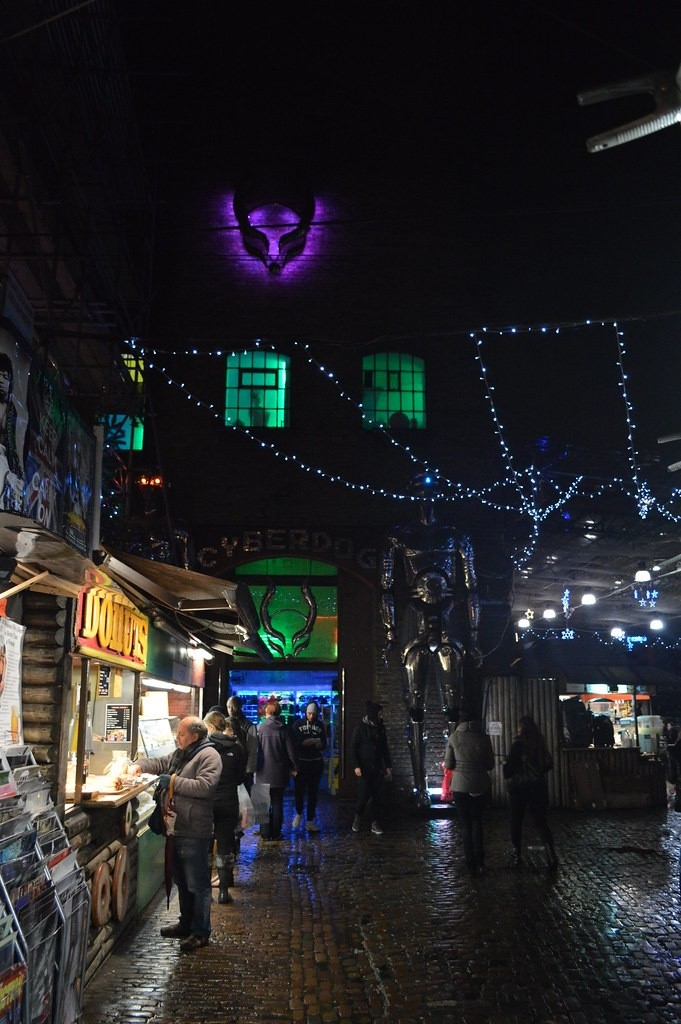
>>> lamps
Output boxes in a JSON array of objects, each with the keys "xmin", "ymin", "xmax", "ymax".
[{"xmin": 517, "ymin": 559, "xmax": 651, "ymax": 629}]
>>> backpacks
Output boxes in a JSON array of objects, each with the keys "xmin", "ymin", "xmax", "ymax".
[{"xmin": 593, "ymin": 716, "xmax": 614, "ymax": 749}]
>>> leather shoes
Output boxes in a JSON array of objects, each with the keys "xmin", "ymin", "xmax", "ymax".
[{"xmin": 160, "ymin": 924, "xmax": 209, "ymax": 950}]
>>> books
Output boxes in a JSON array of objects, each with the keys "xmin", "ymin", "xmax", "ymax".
[{"xmin": 0, "ymin": 764, "xmax": 93, "ymax": 1024}]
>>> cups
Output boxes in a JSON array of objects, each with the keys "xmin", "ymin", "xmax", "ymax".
[{"xmin": 113, "ymin": 751, "xmax": 127, "ymax": 774}]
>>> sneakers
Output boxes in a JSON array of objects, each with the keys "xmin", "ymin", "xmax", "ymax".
[
  {"xmin": 370, "ymin": 822, "xmax": 384, "ymax": 835},
  {"xmin": 305, "ymin": 821, "xmax": 319, "ymax": 831},
  {"xmin": 351, "ymin": 815, "xmax": 360, "ymax": 833},
  {"xmin": 292, "ymin": 814, "xmax": 304, "ymax": 829}
]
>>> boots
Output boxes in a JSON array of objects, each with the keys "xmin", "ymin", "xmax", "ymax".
[
  {"xmin": 216, "ymin": 853, "xmax": 234, "ymax": 905},
  {"xmin": 544, "ymin": 843, "xmax": 558, "ymax": 872},
  {"xmin": 511, "ymin": 845, "xmax": 525, "ymax": 871}
]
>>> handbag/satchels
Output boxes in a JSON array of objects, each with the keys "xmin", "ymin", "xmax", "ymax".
[
  {"xmin": 440, "ymin": 766, "xmax": 454, "ymax": 802},
  {"xmin": 506, "ymin": 744, "xmax": 542, "ymax": 795},
  {"xmin": 255, "ymin": 727, "xmax": 264, "ymax": 774},
  {"xmin": 147, "ymin": 806, "xmax": 168, "ymax": 836},
  {"xmin": 235, "ymin": 783, "xmax": 270, "ymax": 833}
]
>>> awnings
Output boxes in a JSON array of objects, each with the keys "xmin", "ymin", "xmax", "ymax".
[{"xmin": 105, "ymin": 549, "xmax": 241, "ymax": 656}]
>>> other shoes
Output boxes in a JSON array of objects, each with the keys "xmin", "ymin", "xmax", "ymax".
[
  {"xmin": 255, "ymin": 832, "xmax": 281, "ymax": 841},
  {"xmin": 464, "ymin": 858, "xmax": 484, "ymax": 872}
]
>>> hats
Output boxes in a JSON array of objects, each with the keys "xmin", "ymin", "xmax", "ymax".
[
  {"xmin": 307, "ymin": 702, "xmax": 318, "ymax": 713},
  {"xmin": 367, "ymin": 701, "xmax": 383, "ymax": 724}
]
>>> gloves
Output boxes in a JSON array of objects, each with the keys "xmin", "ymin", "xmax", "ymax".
[{"xmin": 158, "ymin": 774, "xmax": 170, "ymax": 789}]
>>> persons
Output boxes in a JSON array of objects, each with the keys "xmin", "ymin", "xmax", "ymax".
[
  {"xmin": 0, "ymin": 352, "xmax": 23, "ymax": 478},
  {"xmin": 292, "ymin": 703, "xmax": 327, "ymax": 832},
  {"xmin": 636, "ymin": 703, "xmax": 642, "ymax": 716},
  {"xmin": 572, "ymin": 714, "xmax": 616, "ymax": 802},
  {"xmin": 503, "ymin": 716, "xmax": 560, "ymax": 866},
  {"xmin": 126, "ymin": 715, "xmax": 223, "ymax": 951},
  {"xmin": 445, "ymin": 708, "xmax": 496, "ymax": 877},
  {"xmin": 255, "ymin": 702, "xmax": 298, "ymax": 846},
  {"xmin": 203, "ymin": 696, "xmax": 257, "ymax": 903},
  {"xmin": 666, "ymin": 721, "xmax": 677, "ymax": 744},
  {"xmin": 666, "ymin": 739, "xmax": 681, "ymax": 814},
  {"xmin": 350, "ymin": 700, "xmax": 393, "ymax": 834}
]
